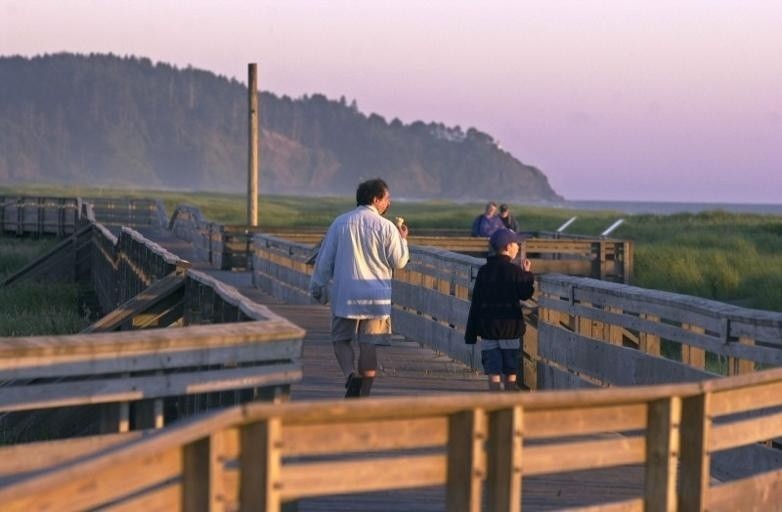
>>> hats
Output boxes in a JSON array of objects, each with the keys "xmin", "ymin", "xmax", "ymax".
[{"xmin": 490, "ymin": 229, "xmax": 525, "ymax": 250}]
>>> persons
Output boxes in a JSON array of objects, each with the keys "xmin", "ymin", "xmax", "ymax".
[
  {"xmin": 309, "ymin": 178, "xmax": 409, "ymax": 399},
  {"xmin": 464, "ymin": 228, "xmax": 534, "ymax": 391},
  {"xmin": 472, "ymin": 201, "xmax": 507, "ymax": 238},
  {"xmin": 497, "ymin": 204, "xmax": 519, "ymax": 232}
]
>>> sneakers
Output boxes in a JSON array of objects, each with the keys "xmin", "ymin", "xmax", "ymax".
[{"xmin": 344, "ymin": 372, "xmax": 363, "ymax": 398}]
